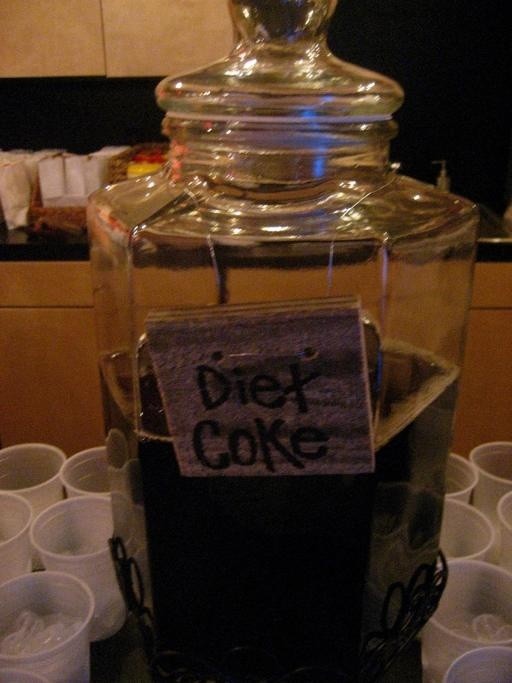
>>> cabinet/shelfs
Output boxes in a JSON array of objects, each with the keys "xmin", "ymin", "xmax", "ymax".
[
  {"xmin": 1, "ymin": 0, "xmax": 245, "ymax": 78},
  {"xmin": 0, "ymin": 261, "xmax": 220, "ymax": 454},
  {"xmin": 220, "ymin": 261, "xmax": 512, "ymax": 442}
]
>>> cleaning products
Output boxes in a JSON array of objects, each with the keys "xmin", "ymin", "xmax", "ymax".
[{"xmin": 432, "ymin": 157, "xmax": 451, "ymax": 190}]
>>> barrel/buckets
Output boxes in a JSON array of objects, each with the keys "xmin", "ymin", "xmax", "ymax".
[
  {"xmin": 0, "ymin": 441, "xmax": 126, "ymax": 682},
  {"xmin": 425, "ymin": 441, "xmax": 512, "ymax": 683}
]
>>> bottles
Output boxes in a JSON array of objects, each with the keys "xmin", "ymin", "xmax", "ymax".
[{"xmin": 94, "ymin": 0, "xmax": 479, "ymax": 682}]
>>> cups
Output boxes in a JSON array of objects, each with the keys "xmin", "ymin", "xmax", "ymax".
[
  {"xmin": 422, "ymin": 440, "xmax": 512, "ymax": 683},
  {"xmin": 0, "ymin": 439, "xmax": 148, "ymax": 683}
]
